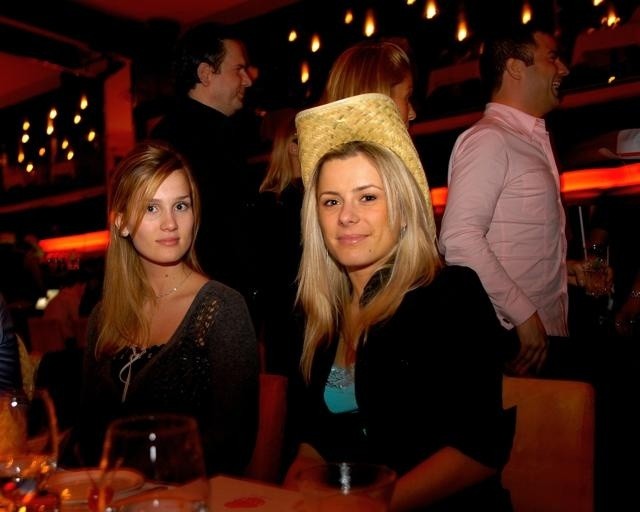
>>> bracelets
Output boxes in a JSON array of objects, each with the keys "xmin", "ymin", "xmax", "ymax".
[{"xmin": 629, "ymin": 287, "xmax": 639, "ymax": 300}]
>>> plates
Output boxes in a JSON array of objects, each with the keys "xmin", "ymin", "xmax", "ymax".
[{"xmin": 36, "ymin": 468, "xmax": 146, "ymax": 508}]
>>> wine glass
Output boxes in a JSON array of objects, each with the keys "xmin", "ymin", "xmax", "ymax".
[{"xmin": 0, "ymin": 388, "xmax": 61, "ymax": 511}]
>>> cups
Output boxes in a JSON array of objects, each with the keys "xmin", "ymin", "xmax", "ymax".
[
  {"xmin": 584, "ymin": 240, "xmax": 614, "ymax": 299},
  {"xmin": 98, "ymin": 414, "xmax": 212, "ymax": 512}
]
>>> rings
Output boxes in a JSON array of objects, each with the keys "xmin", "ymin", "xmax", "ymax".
[
  {"xmin": 626, "ymin": 319, "xmax": 636, "ymax": 324},
  {"xmin": 616, "ymin": 319, "xmax": 622, "ymax": 330}
]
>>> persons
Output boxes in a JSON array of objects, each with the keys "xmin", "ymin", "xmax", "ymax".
[
  {"xmin": 585, "ymin": 185, "xmax": 620, "ymax": 256},
  {"xmin": 41, "ymin": 135, "xmax": 262, "ymax": 482},
  {"xmin": 314, "ymin": 36, "xmax": 423, "ymax": 132},
  {"xmin": 435, "ymin": 20, "xmax": 616, "ymax": 378},
  {"xmin": 1, "ymin": 229, "xmax": 104, "ymax": 443},
  {"xmin": 280, "ymin": 140, "xmax": 516, "ymax": 511},
  {"xmin": 145, "ymin": 18, "xmax": 306, "ymax": 372},
  {"xmin": 615, "ymin": 269, "xmax": 640, "ymax": 341}
]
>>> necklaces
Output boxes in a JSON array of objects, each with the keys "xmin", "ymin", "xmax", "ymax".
[{"xmin": 139, "ymin": 267, "xmax": 195, "ymax": 300}]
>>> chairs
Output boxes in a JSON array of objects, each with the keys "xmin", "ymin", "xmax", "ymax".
[
  {"xmin": 502, "ymin": 377, "xmax": 595, "ymax": 510},
  {"xmin": 247, "ymin": 374, "xmax": 289, "ymax": 483}
]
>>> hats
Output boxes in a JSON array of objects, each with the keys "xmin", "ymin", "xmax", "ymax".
[{"xmin": 296, "ymin": 94, "xmax": 433, "ymax": 218}]
